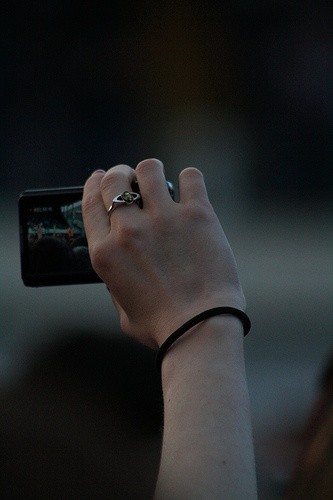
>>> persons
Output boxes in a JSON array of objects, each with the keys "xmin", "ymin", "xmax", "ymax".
[{"xmin": 79, "ymin": 157, "xmax": 333, "ymax": 500}]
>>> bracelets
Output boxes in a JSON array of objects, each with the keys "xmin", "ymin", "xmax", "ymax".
[{"xmin": 153, "ymin": 306, "xmax": 251, "ymax": 377}]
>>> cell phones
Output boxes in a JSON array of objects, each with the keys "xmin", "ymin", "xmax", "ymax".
[{"xmin": 19, "ymin": 182, "xmax": 174, "ymax": 287}]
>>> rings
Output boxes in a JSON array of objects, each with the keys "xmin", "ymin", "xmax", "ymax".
[{"xmin": 106, "ymin": 190, "xmax": 141, "ymax": 216}]
